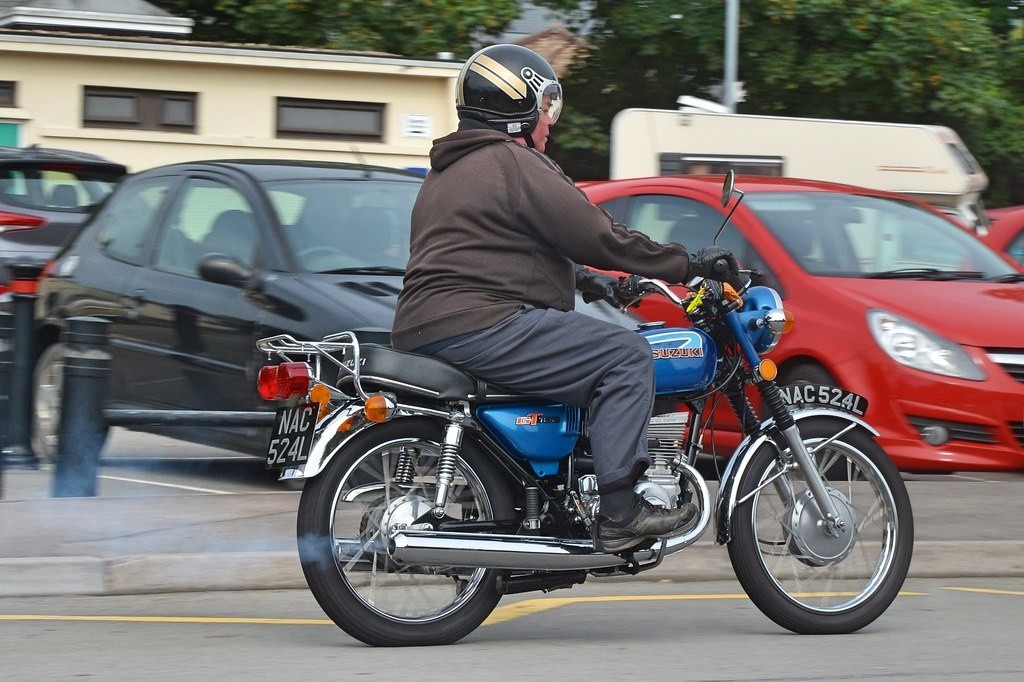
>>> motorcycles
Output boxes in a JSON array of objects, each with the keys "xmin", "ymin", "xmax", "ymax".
[{"xmin": 248, "ymin": 242, "xmax": 916, "ymax": 648}]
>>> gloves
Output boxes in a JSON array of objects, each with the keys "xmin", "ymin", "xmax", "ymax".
[
  {"xmin": 683, "ymin": 245, "xmax": 742, "ymax": 285},
  {"xmin": 582, "ymin": 270, "xmax": 654, "ymax": 312}
]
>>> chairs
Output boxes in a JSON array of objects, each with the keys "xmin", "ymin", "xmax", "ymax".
[{"xmin": 207, "ymin": 211, "xmax": 255, "ymax": 274}]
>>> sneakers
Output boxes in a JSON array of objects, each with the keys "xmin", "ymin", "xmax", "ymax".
[{"xmin": 597, "ymin": 492, "xmax": 699, "ymax": 554}]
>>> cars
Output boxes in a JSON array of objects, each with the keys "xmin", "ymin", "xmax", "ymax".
[
  {"xmin": 33, "ymin": 163, "xmax": 654, "ymax": 492},
  {"xmin": 569, "ymin": 170, "xmax": 1024, "ymax": 478},
  {"xmin": 957, "ymin": 207, "xmax": 1024, "ymax": 279}
]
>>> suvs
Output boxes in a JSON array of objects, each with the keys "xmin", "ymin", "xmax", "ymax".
[{"xmin": 1, "ymin": 140, "xmax": 140, "ymax": 464}]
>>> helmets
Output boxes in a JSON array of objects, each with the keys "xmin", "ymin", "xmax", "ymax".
[{"xmin": 456, "ymin": 43, "xmax": 564, "ymax": 138}]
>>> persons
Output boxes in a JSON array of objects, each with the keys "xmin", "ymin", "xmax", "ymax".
[{"xmin": 390, "ymin": 44, "xmax": 743, "ymax": 554}]
